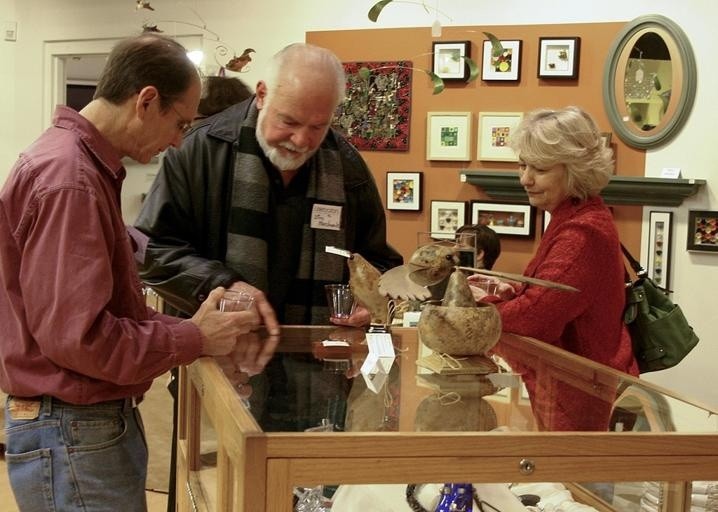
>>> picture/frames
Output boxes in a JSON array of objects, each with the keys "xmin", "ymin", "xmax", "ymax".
[
  {"xmin": 647, "ymin": 210, "xmax": 673, "ymax": 296},
  {"xmin": 476, "ymin": 111, "xmax": 524, "ymax": 165},
  {"xmin": 428, "ymin": 199, "xmax": 469, "ymax": 239},
  {"xmin": 429, "ymin": 39, "xmax": 472, "ymax": 83},
  {"xmin": 425, "ymin": 110, "xmax": 472, "ymax": 163},
  {"xmin": 541, "ymin": 210, "xmax": 553, "ymax": 237},
  {"xmin": 599, "ymin": 130, "xmax": 618, "ymax": 177},
  {"xmin": 384, "ymin": 169, "xmax": 424, "ymax": 212},
  {"xmin": 684, "ymin": 207, "xmax": 718, "ymax": 254},
  {"xmin": 472, "ymin": 199, "xmax": 536, "ymax": 240},
  {"xmin": 481, "ymin": 39, "xmax": 524, "ymax": 84},
  {"xmin": 536, "ymin": 35, "xmax": 581, "ymax": 82}
]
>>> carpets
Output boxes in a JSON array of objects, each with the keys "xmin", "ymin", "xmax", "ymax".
[{"xmin": 0, "ymin": 378, "xmax": 174, "ymax": 492}]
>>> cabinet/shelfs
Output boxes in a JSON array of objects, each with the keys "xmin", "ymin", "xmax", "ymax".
[{"xmin": 172, "ymin": 320, "xmax": 717, "ymax": 510}]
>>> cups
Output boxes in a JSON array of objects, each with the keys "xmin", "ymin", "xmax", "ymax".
[
  {"xmin": 218, "ymin": 288, "xmax": 256, "ymax": 314},
  {"xmin": 323, "ymin": 339, "xmax": 353, "ymax": 372},
  {"xmin": 324, "ymin": 283, "xmax": 357, "ymax": 319}
]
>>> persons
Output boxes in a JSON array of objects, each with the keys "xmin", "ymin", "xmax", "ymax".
[
  {"xmin": 130, "ymin": 43, "xmax": 403, "ymax": 512},
  {"xmin": 232, "ymin": 333, "xmax": 279, "ymax": 377},
  {"xmin": 475, "ymin": 104, "xmax": 641, "ymax": 503},
  {"xmin": 0, "ymin": 30, "xmax": 258, "ymax": 512},
  {"xmin": 450, "ymin": 223, "xmax": 500, "ymax": 293}
]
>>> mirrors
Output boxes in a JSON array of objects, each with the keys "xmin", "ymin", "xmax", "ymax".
[{"xmin": 601, "ymin": 13, "xmax": 698, "ymax": 153}]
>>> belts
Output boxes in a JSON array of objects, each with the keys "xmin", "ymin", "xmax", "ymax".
[{"xmin": 35, "ymin": 395, "xmax": 146, "ymax": 409}]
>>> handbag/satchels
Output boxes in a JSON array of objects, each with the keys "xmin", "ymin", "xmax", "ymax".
[{"xmin": 625, "ymin": 263, "xmax": 699, "ymax": 373}]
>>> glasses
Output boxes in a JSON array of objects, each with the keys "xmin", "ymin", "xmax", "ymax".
[{"xmin": 162, "ymin": 96, "xmax": 194, "ymax": 138}]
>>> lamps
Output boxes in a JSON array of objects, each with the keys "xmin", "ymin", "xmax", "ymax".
[
  {"xmin": 624, "ymin": 48, "xmax": 664, "ymax": 101},
  {"xmin": 135, "ymin": 1, "xmax": 252, "ymax": 100}
]
